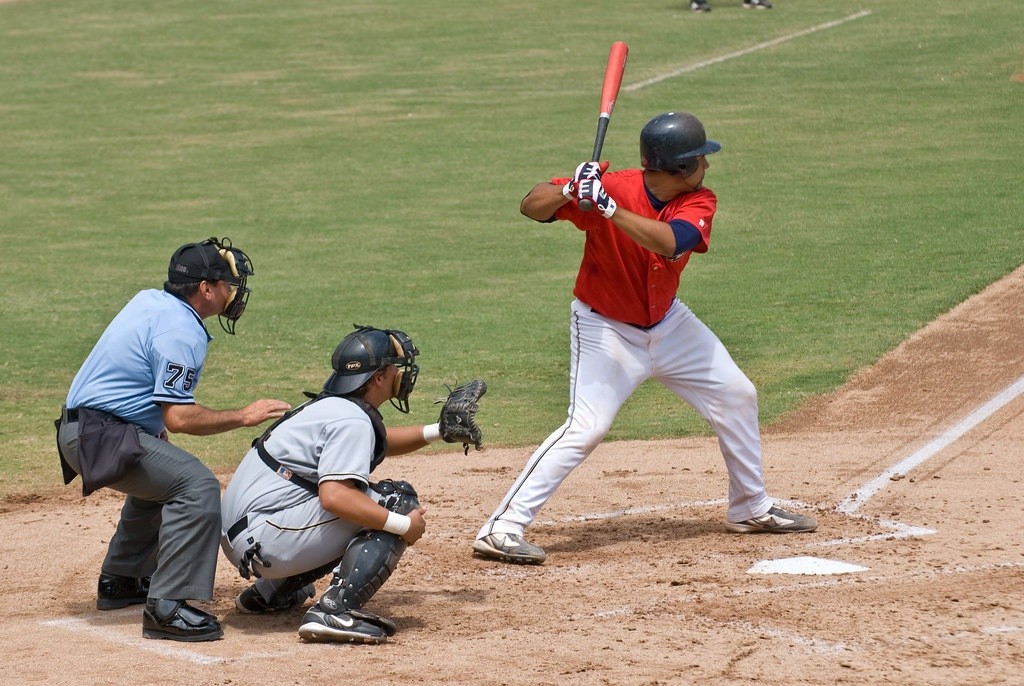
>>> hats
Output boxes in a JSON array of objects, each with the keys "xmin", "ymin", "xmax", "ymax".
[{"xmin": 168, "ymin": 241, "xmax": 236, "ymax": 282}]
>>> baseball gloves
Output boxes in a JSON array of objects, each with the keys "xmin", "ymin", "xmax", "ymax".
[{"xmin": 437, "ymin": 379, "xmax": 489, "ymax": 448}]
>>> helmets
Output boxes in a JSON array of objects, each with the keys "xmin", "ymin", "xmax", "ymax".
[
  {"xmin": 640, "ymin": 112, "xmax": 722, "ymax": 179},
  {"xmin": 321, "ymin": 330, "xmax": 395, "ymax": 395}
]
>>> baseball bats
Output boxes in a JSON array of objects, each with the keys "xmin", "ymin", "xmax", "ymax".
[{"xmin": 591, "ymin": 41, "xmax": 629, "ymax": 162}]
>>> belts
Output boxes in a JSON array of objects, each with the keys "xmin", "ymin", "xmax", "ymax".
[
  {"xmin": 227, "ymin": 516, "xmax": 248, "ymax": 542},
  {"xmin": 61, "ymin": 406, "xmax": 79, "ymax": 422},
  {"xmin": 590, "ymin": 308, "xmax": 661, "ymax": 329}
]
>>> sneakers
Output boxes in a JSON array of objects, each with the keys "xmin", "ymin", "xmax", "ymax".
[
  {"xmin": 235, "ymin": 582, "xmax": 315, "ymax": 614},
  {"xmin": 472, "ymin": 533, "xmax": 545, "ymax": 565},
  {"xmin": 298, "ymin": 606, "xmax": 387, "ymax": 644},
  {"xmin": 725, "ymin": 503, "xmax": 818, "ymax": 533}
]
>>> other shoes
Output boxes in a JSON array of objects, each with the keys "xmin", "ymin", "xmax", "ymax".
[
  {"xmin": 743, "ymin": 0, "xmax": 772, "ymax": 9},
  {"xmin": 689, "ymin": 0, "xmax": 710, "ymax": 12}
]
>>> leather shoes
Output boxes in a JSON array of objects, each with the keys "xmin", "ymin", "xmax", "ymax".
[
  {"xmin": 142, "ymin": 604, "xmax": 224, "ymax": 642},
  {"xmin": 96, "ymin": 574, "xmax": 153, "ymax": 610}
]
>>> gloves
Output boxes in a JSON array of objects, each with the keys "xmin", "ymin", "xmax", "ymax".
[
  {"xmin": 562, "ymin": 160, "xmax": 611, "ymax": 201},
  {"xmin": 577, "ymin": 179, "xmax": 617, "ymax": 219}
]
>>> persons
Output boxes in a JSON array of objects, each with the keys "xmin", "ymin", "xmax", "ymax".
[
  {"xmin": 474, "ymin": 111, "xmax": 821, "ymax": 566},
  {"xmin": 54, "ymin": 238, "xmax": 292, "ymax": 644},
  {"xmin": 220, "ymin": 321, "xmax": 488, "ymax": 646},
  {"xmin": 686, "ymin": 0, "xmax": 771, "ymax": 14}
]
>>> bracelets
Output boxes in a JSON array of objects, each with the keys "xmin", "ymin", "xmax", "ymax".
[
  {"xmin": 422, "ymin": 423, "xmax": 442, "ymax": 444},
  {"xmin": 383, "ymin": 511, "xmax": 411, "ymax": 535}
]
